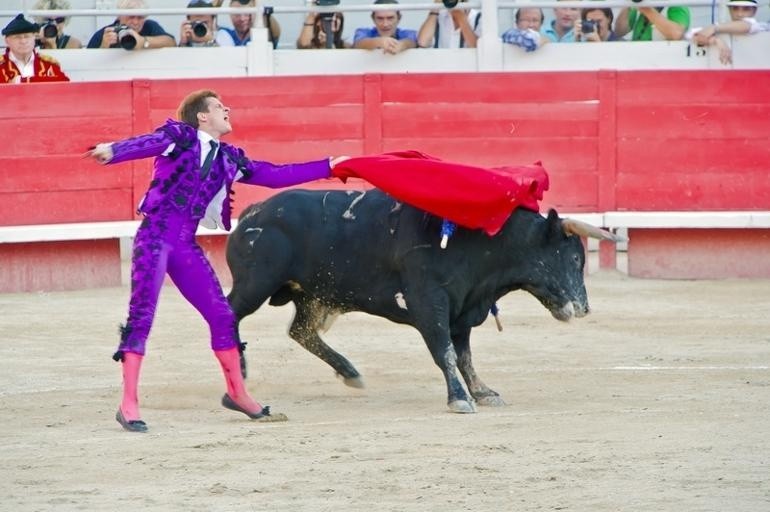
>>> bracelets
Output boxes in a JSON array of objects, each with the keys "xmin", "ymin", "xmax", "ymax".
[{"xmin": 714, "ymin": 23, "xmax": 719, "ymax": 35}]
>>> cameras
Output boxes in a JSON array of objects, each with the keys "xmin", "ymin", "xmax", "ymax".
[
  {"xmin": 442, "ymin": 0, "xmax": 457, "ymax": 9},
  {"xmin": 44, "ymin": 23, "xmax": 58, "ymax": 38},
  {"xmin": 582, "ymin": 19, "xmax": 600, "ymax": 33},
  {"xmin": 317, "ymin": 0, "xmax": 340, "ymax": 19},
  {"xmin": 114, "ymin": 25, "xmax": 136, "ymax": 51},
  {"xmin": 190, "ymin": 20, "xmax": 208, "ymax": 38}
]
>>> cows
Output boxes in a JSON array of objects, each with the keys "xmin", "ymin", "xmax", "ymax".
[{"xmin": 223, "ymin": 187, "xmax": 629, "ymax": 415}]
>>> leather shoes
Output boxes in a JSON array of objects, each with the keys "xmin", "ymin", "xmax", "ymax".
[
  {"xmin": 221, "ymin": 394, "xmax": 270, "ymax": 420},
  {"xmin": 115, "ymin": 406, "xmax": 148, "ymax": 433}
]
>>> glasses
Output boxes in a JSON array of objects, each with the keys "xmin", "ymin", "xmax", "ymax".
[
  {"xmin": 189, "ymin": 0, "xmax": 211, "ymax": 5},
  {"xmin": 517, "ymin": 17, "xmax": 540, "ymax": 25},
  {"xmin": 122, "ymin": 15, "xmax": 146, "ymax": 22},
  {"xmin": 43, "ymin": 16, "xmax": 66, "ymax": 25}
]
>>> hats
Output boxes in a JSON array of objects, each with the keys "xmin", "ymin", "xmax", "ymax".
[
  {"xmin": 725, "ymin": 0, "xmax": 758, "ymax": 8},
  {"xmin": 1, "ymin": 14, "xmax": 40, "ymax": 35}
]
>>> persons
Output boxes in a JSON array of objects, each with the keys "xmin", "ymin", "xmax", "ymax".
[
  {"xmin": 79, "ymin": 87, "xmax": 354, "ymax": 434},
  {"xmin": 27, "ymin": 1, "xmax": 81, "ymax": 49},
  {"xmin": 178, "ymin": 1, "xmax": 281, "ymax": 50},
  {"xmin": 1, "ymin": 13, "xmax": 70, "ymax": 84},
  {"xmin": 86, "ymin": 2, "xmax": 177, "ymax": 49},
  {"xmin": 684, "ymin": 1, "xmax": 770, "ymax": 66},
  {"xmin": 502, "ymin": 1, "xmax": 690, "ymax": 52},
  {"xmin": 295, "ymin": 0, "xmax": 483, "ymax": 55}
]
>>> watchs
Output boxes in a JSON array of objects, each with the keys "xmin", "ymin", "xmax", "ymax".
[{"xmin": 143, "ymin": 36, "xmax": 150, "ymax": 49}]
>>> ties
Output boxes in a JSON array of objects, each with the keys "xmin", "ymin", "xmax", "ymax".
[{"xmin": 199, "ymin": 139, "xmax": 219, "ymax": 182}]
